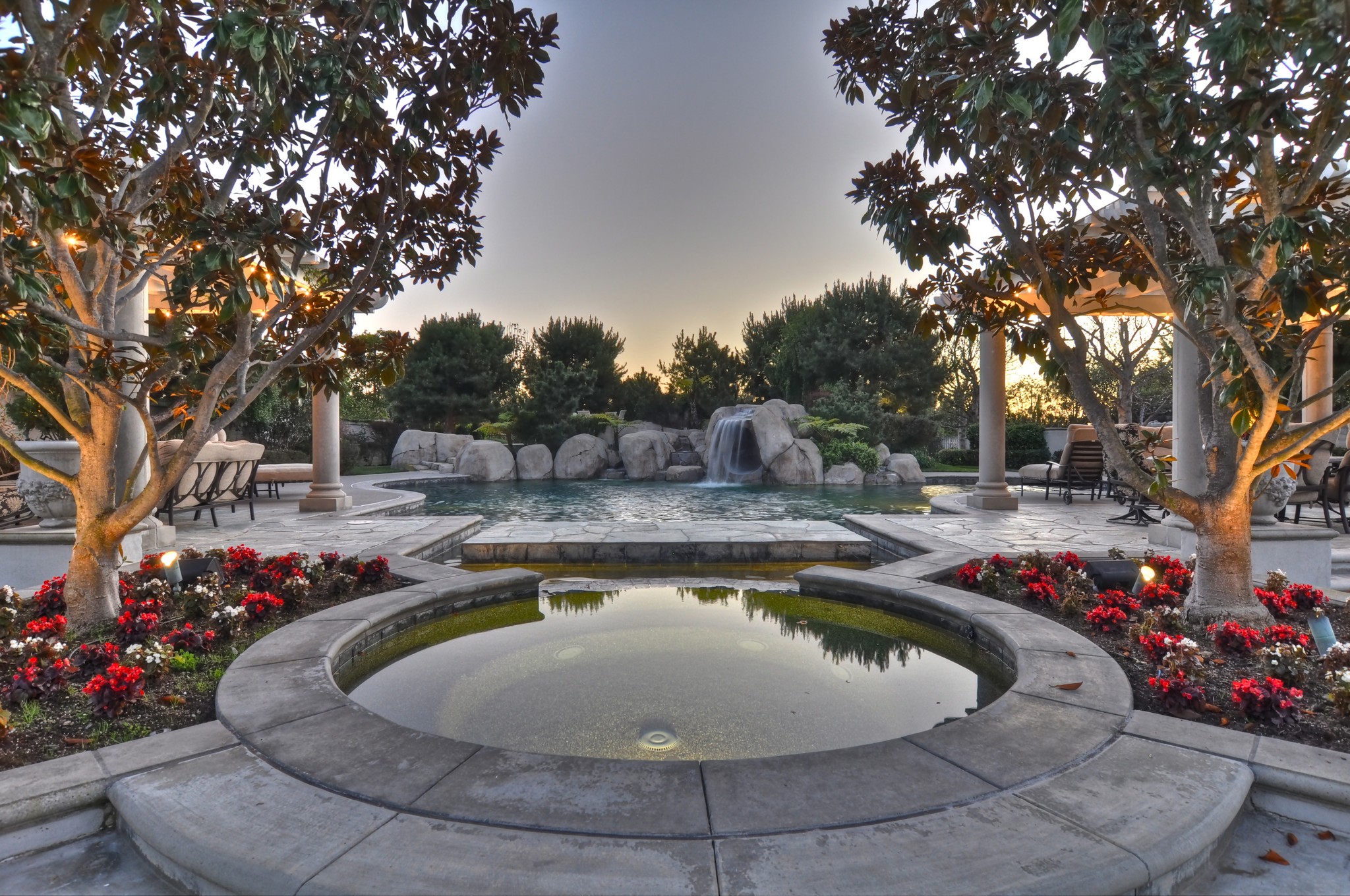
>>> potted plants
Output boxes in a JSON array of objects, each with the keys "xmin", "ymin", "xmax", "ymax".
[
  {"xmin": 1, "ymin": 309, "xmax": 85, "ymax": 531},
  {"xmin": 1235, "ymin": 337, "xmax": 1304, "ymax": 524}
]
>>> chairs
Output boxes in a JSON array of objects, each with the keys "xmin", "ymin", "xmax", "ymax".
[
  {"xmin": 1278, "ymin": 421, "xmax": 1340, "ymax": 531},
  {"xmin": 1019, "ymin": 423, "xmax": 1109, "ymax": 505},
  {"xmin": 1294, "ymin": 423, "xmax": 1350, "ymax": 533},
  {"xmin": 1103, "ymin": 423, "xmax": 1178, "ymax": 529}
]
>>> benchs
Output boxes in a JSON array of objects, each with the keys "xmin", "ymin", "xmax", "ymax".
[
  {"xmin": 147, "ymin": 439, "xmax": 266, "ymax": 530},
  {"xmin": 249, "ymin": 462, "xmax": 318, "ymax": 499}
]
